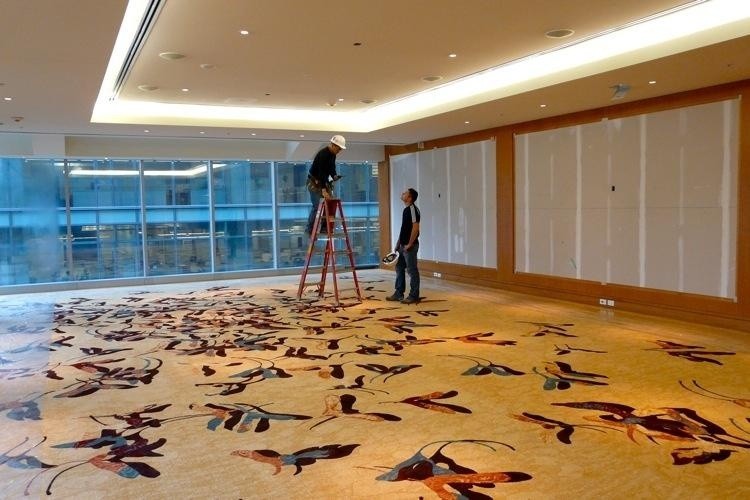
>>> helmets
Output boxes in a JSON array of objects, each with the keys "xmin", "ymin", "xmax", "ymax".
[
  {"xmin": 382, "ymin": 251, "xmax": 400, "ymax": 267},
  {"xmin": 331, "ymin": 135, "xmax": 347, "ymax": 150}
]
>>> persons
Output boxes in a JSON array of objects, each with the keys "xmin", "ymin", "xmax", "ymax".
[
  {"xmin": 386, "ymin": 188, "xmax": 420, "ymax": 304},
  {"xmin": 305, "ymin": 135, "xmax": 346, "ymax": 234}
]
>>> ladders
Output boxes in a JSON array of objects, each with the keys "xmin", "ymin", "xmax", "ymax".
[{"xmin": 296, "ymin": 197, "xmax": 363, "ymax": 307}]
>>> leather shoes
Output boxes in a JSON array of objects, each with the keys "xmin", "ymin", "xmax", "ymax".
[
  {"xmin": 385, "ymin": 291, "xmax": 404, "ymax": 301},
  {"xmin": 400, "ymin": 297, "xmax": 420, "ymax": 304}
]
